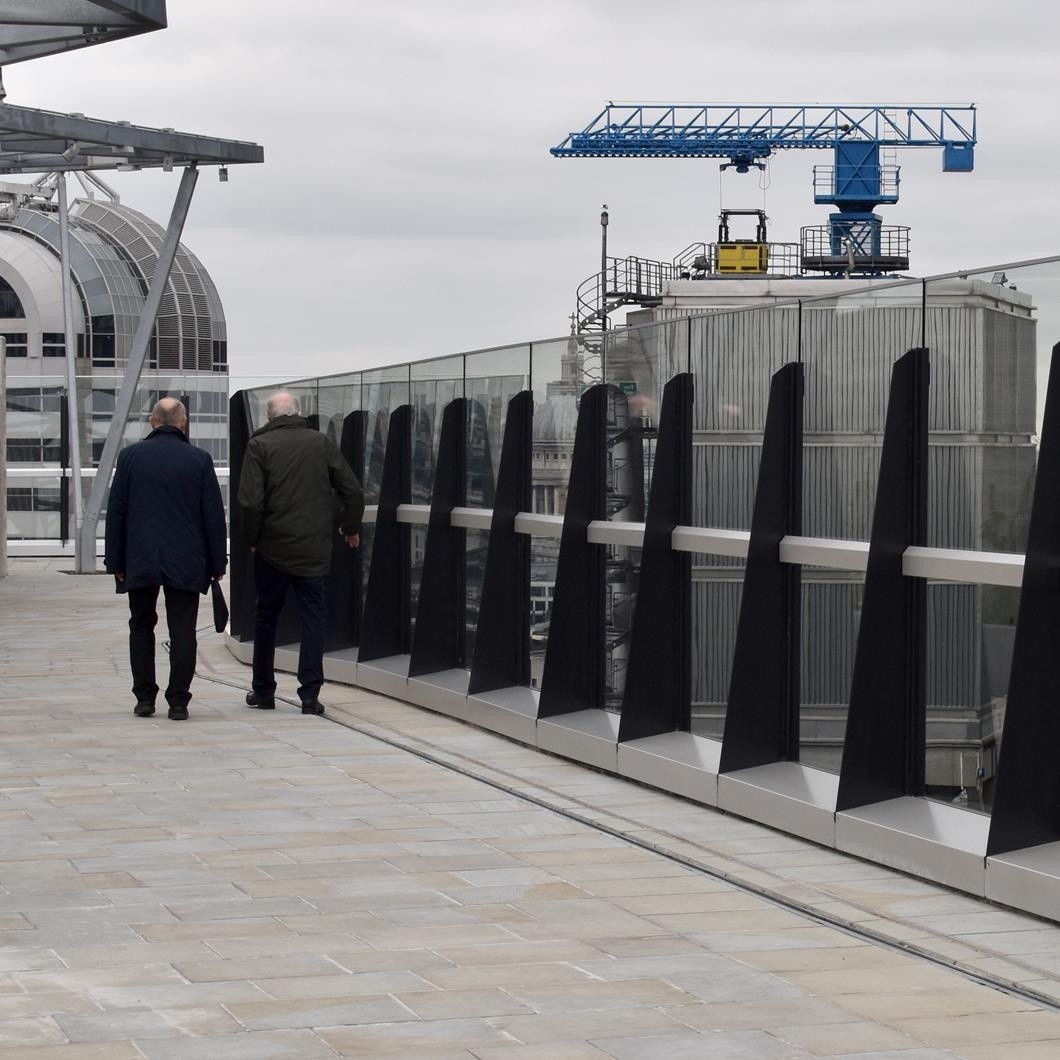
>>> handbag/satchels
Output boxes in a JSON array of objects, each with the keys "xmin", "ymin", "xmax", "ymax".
[{"xmin": 210, "ymin": 576, "xmax": 229, "ymax": 634}]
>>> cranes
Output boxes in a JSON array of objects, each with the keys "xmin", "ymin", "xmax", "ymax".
[{"xmin": 548, "ymin": 100, "xmax": 978, "ymax": 256}]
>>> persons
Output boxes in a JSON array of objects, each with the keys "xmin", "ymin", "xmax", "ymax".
[
  {"xmin": 104, "ymin": 397, "xmax": 228, "ymax": 721},
  {"xmin": 237, "ymin": 386, "xmax": 366, "ymax": 714}
]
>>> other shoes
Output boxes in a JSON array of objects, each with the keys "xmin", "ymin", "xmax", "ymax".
[
  {"xmin": 246, "ymin": 691, "xmax": 276, "ymax": 710},
  {"xmin": 168, "ymin": 708, "xmax": 188, "ymax": 720},
  {"xmin": 302, "ymin": 700, "xmax": 325, "ymax": 714},
  {"xmin": 134, "ymin": 702, "xmax": 155, "ymax": 714}
]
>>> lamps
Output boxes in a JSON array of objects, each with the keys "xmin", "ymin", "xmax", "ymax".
[
  {"xmin": 991, "ymin": 272, "xmax": 1008, "ymax": 287},
  {"xmin": 60, "ymin": 141, "xmax": 79, "ymax": 162}
]
